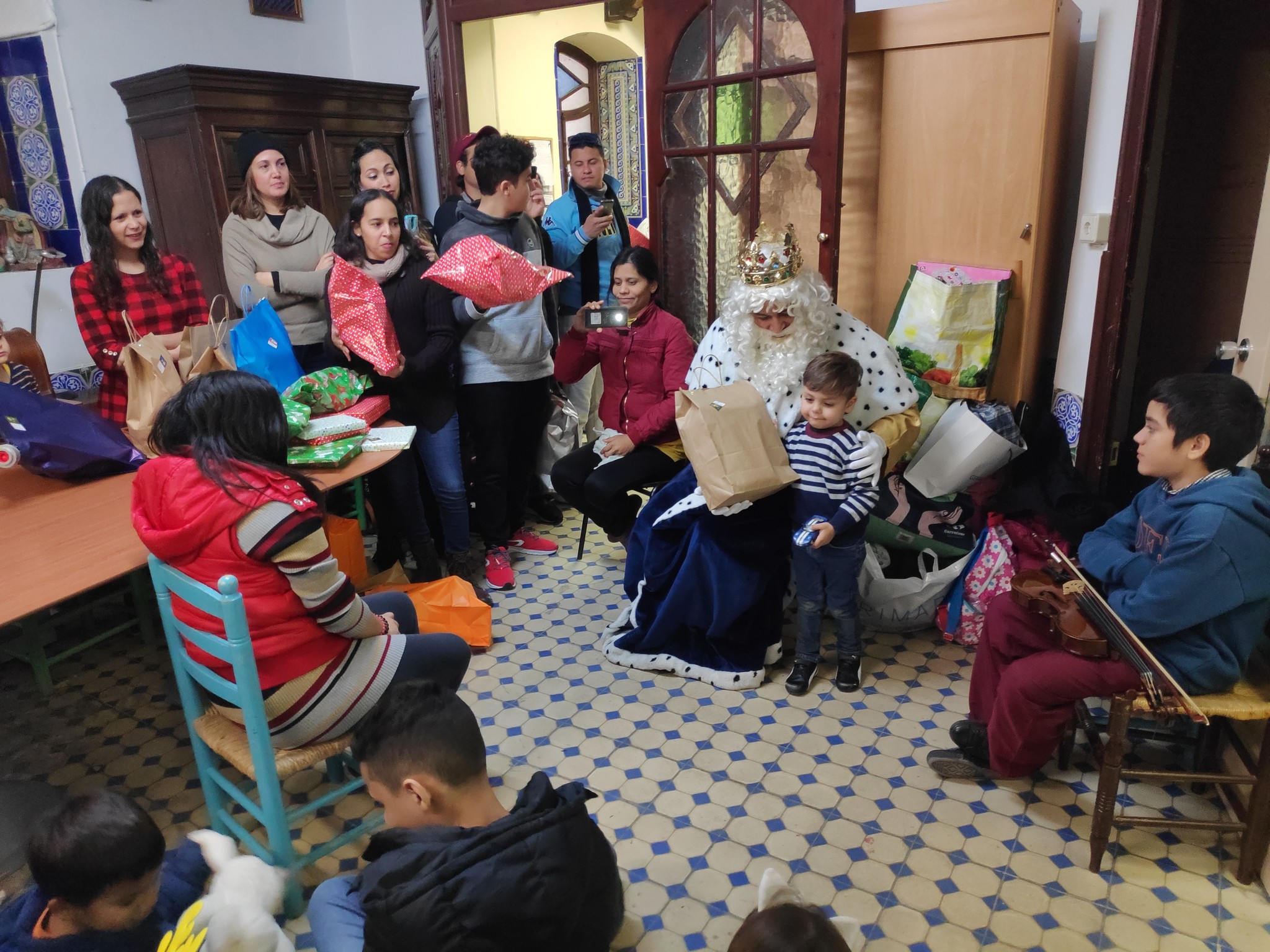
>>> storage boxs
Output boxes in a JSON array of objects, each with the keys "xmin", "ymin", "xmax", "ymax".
[{"xmin": 287, "ymin": 395, "xmax": 391, "ymax": 468}]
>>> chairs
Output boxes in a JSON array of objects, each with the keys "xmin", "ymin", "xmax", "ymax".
[
  {"xmin": 146, "ymin": 552, "xmax": 386, "ymax": 919},
  {"xmin": 577, "ymin": 334, "xmax": 700, "ymax": 560}
]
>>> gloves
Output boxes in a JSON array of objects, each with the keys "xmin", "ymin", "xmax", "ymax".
[
  {"xmin": 694, "ymin": 485, "xmax": 753, "ymax": 516},
  {"xmin": 849, "ymin": 430, "xmax": 889, "ymax": 487}
]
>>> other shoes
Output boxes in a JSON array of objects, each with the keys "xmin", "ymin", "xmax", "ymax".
[
  {"xmin": 526, "ymin": 495, "xmax": 564, "ymax": 525},
  {"xmin": 784, "ymin": 657, "xmax": 819, "ymax": 696},
  {"xmin": 834, "ymin": 654, "xmax": 863, "ymax": 693},
  {"xmin": 602, "ymin": 493, "xmax": 641, "ymax": 542},
  {"xmin": 926, "ymin": 717, "xmax": 994, "ymax": 781},
  {"xmin": 370, "ymin": 538, "xmax": 407, "ymax": 576}
]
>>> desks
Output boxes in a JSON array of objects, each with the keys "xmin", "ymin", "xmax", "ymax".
[{"xmin": 0, "ymin": 417, "xmax": 405, "ymax": 691}]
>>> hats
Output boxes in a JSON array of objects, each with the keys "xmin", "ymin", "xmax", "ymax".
[
  {"xmin": 447, "ymin": 125, "xmax": 500, "ymax": 180},
  {"xmin": 234, "ymin": 128, "xmax": 282, "ymax": 176}
]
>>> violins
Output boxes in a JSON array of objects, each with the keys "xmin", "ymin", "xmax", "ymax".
[{"xmin": 1009, "ymin": 557, "xmax": 1182, "ymax": 727}]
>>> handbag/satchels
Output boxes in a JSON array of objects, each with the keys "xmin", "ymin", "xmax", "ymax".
[
  {"xmin": 886, "ymin": 261, "xmax": 1015, "ymax": 405},
  {"xmin": 675, "ymin": 355, "xmax": 800, "ymax": 509},
  {"xmin": 853, "ymin": 373, "xmax": 1090, "ymax": 644},
  {"xmin": 119, "ymin": 308, "xmax": 186, "ymax": 459},
  {"xmin": 176, "ymin": 293, "xmax": 246, "ymax": 384},
  {"xmin": 538, "ymin": 393, "xmax": 580, "ymax": 474},
  {"xmin": 229, "ymin": 284, "xmax": 305, "ymax": 397}
]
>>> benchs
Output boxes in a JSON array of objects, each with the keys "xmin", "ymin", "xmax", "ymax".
[{"xmin": 1058, "ymin": 669, "xmax": 1270, "ymax": 886}]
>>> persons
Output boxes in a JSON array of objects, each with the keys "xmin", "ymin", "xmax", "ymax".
[
  {"xmin": 780, "ymin": 356, "xmax": 879, "ymax": 697},
  {"xmin": 222, "ymin": 128, "xmax": 337, "ymax": 376},
  {"xmin": 0, "ymin": 319, "xmax": 40, "ymax": 394},
  {"xmin": 0, "ymin": 372, "xmax": 860, "ymax": 952},
  {"xmin": 323, "ymin": 125, "xmax": 631, "ymax": 590},
  {"xmin": 70, "ymin": 174, "xmax": 212, "ymax": 429},
  {"xmin": 551, "ymin": 246, "xmax": 695, "ymax": 551},
  {"xmin": 601, "ymin": 217, "xmax": 920, "ymax": 687},
  {"xmin": 925, "ymin": 372, "xmax": 1270, "ymax": 781}
]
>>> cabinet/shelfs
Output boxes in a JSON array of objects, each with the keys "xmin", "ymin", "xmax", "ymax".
[{"xmin": 109, "ymin": 64, "xmax": 422, "ymax": 324}]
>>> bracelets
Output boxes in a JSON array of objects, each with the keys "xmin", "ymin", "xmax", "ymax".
[
  {"xmin": 378, "ymin": 614, "xmax": 389, "ymax": 635},
  {"xmin": 376, "ymin": 614, "xmax": 386, "ymax": 635}
]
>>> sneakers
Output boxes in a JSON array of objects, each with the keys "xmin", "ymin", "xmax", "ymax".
[
  {"xmin": 507, "ymin": 526, "xmax": 559, "ymax": 556},
  {"xmin": 442, "ymin": 551, "xmax": 494, "ymax": 609},
  {"xmin": 484, "ymin": 542, "xmax": 517, "ymax": 591},
  {"xmin": 410, "ymin": 542, "xmax": 441, "ymax": 584}
]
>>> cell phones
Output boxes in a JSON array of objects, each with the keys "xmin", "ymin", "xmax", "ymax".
[
  {"xmin": 404, "ymin": 216, "xmax": 419, "ymax": 233},
  {"xmin": 584, "ymin": 306, "xmax": 629, "ymax": 328},
  {"xmin": 530, "ymin": 166, "xmax": 537, "ymax": 179},
  {"xmin": 599, "ymin": 200, "xmax": 614, "ymax": 218}
]
